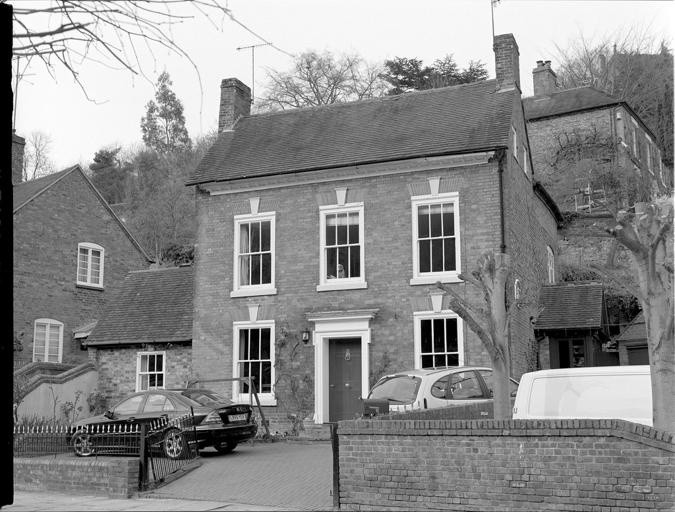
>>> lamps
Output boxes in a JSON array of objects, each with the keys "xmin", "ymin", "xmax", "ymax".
[{"xmin": 302, "ymin": 328, "xmax": 310, "ymax": 345}]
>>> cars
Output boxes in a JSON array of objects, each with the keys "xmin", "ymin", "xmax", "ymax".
[{"xmin": 65, "ymin": 387, "xmax": 258, "ymax": 460}]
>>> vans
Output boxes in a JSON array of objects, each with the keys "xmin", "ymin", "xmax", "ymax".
[{"xmin": 362, "ymin": 361, "xmax": 523, "ymax": 420}]
